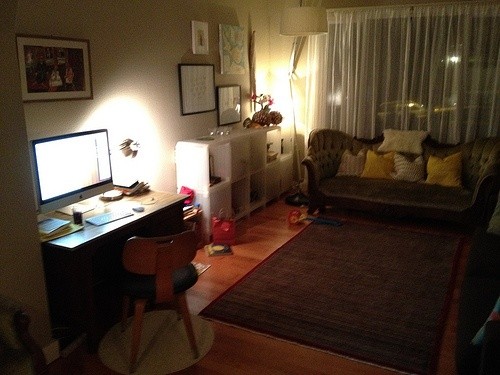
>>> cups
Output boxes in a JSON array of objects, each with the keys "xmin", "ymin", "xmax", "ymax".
[
  {"xmin": 208, "ymin": 127, "xmax": 232, "ymax": 137},
  {"xmin": 72, "ymin": 207, "xmax": 83, "ymax": 226}
]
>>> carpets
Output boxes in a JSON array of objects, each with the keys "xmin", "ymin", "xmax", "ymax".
[
  {"xmin": 98, "ymin": 311, "xmax": 215, "ymax": 375},
  {"xmin": 198, "ymin": 217, "xmax": 462, "ymax": 375}
]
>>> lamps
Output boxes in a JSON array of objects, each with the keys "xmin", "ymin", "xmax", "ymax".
[{"xmin": 279, "ymin": 6, "xmax": 328, "ymax": 73}]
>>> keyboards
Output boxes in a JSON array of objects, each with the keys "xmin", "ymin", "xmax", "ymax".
[{"xmin": 85, "ymin": 209, "xmax": 133, "ymax": 225}]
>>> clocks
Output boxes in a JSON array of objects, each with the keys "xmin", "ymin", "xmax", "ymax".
[{"xmin": 192, "ymin": 21, "xmax": 209, "ymax": 55}]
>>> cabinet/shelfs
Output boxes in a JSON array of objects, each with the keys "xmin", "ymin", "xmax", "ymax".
[{"xmin": 177, "ymin": 122, "xmax": 298, "ymax": 247}]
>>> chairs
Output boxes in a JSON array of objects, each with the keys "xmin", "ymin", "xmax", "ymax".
[{"xmin": 121, "ymin": 229, "xmax": 199, "ymax": 373}]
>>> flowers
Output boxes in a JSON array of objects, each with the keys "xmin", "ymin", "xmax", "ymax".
[{"xmin": 252, "ymin": 93, "xmax": 273, "ymax": 109}]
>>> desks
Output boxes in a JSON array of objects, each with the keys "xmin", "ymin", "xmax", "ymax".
[{"xmin": 40, "ymin": 188, "xmax": 190, "ymax": 353}]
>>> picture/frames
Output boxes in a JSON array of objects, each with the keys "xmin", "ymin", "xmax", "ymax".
[
  {"xmin": 179, "ymin": 64, "xmax": 216, "ymax": 116},
  {"xmin": 15, "ymin": 33, "xmax": 94, "ymax": 102},
  {"xmin": 217, "ymin": 84, "xmax": 241, "ymax": 125}
]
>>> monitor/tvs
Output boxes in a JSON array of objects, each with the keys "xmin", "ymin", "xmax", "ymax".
[{"xmin": 28, "ymin": 129, "xmax": 113, "ymax": 215}]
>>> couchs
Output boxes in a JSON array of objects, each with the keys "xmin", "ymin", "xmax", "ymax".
[
  {"xmin": 457, "ymin": 233, "xmax": 500, "ymax": 375},
  {"xmin": 301, "ymin": 128, "xmax": 500, "ymax": 229}
]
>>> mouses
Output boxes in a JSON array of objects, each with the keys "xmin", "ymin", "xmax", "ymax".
[{"xmin": 132, "ymin": 206, "xmax": 144, "ymax": 212}]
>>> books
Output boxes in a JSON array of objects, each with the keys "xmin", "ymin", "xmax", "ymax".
[
  {"xmin": 113, "ymin": 178, "xmax": 150, "ymax": 196},
  {"xmin": 36, "ymin": 212, "xmax": 71, "ymax": 236}
]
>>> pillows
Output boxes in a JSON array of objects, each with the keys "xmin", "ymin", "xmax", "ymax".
[
  {"xmin": 469, "ymin": 297, "xmax": 500, "ymax": 345},
  {"xmin": 336, "ymin": 128, "xmax": 465, "ymax": 188}
]
